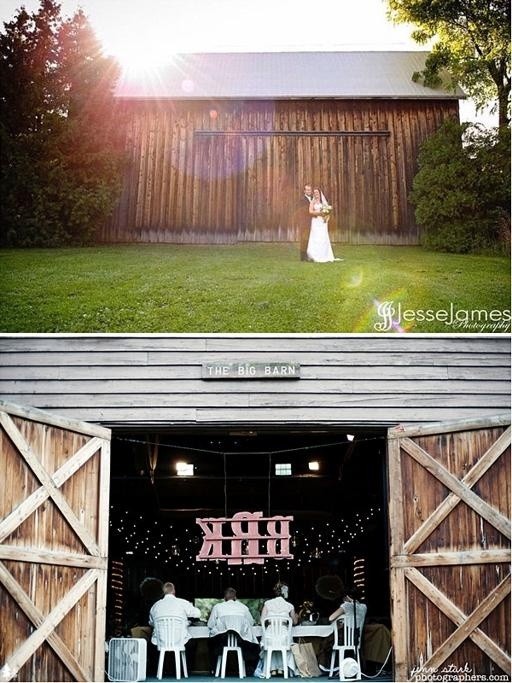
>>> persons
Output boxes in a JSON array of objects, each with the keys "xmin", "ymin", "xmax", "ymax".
[
  {"xmin": 297, "ymin": 184, "xmax": 315, "ymax": 261},
  {"xmin": 205, "ymin": 585, "xmax": 258, "ymax": 675},
  {"xmin": 149, "ymin": 582, "xmax": 201, "ymax": 672},
  {"xmin": 308, "ymin": 187, "xmax": 337, "ymax": 263},
  {"xmin": 254, "ymin": 582, "xmax": 298, "ymax": 678},
  {"xmin": 328, "ymin": 589, "xmax": 368, "ymax": 677}
]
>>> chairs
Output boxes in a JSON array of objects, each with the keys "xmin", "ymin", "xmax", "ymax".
[
  {"xmin": 260, "ymin": 615, "xmax": 293, "ymax": 681},
  {"xmin": 329, "ymin": 615, "xmax": 365, "ymax": 682},
  {"xmin": 157, "ymin": 616, "xmax": 188, "ymax": 680},
  {"xmin": 219, "ymin": 616, "xmax": 251, "ymax": 680}
]
